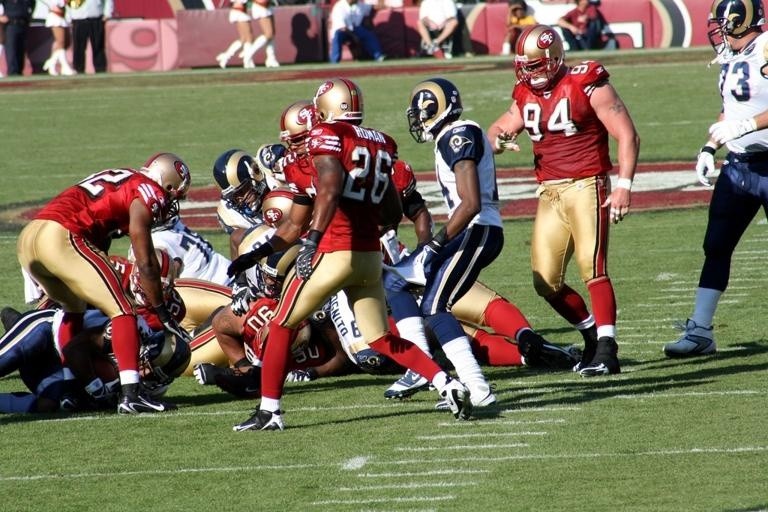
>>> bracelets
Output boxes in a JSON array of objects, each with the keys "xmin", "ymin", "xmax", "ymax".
[
  {"xmin": 700, "ymin": 145, "xmax": 716, "ymax": 155},
  {"xmin": 615, "ymin": 178, "xmax": 632, "ymax": 190}
]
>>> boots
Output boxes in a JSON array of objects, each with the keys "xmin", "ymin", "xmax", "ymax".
[
  {"xmin": 42, "ymin": 48, "xmax": 78, "ymax": 76},
  {"xmin": 215, "ymin": 34, "xmax": 281, "ymax": 69}
]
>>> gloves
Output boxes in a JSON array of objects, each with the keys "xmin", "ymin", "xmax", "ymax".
[
  {"xmin": 193, "ymin": 362, "xmax": 234, "ymax": 386},
  {"xmin": 285, "ymin": 367, "xmax": 317, "ymax": 382},
  {"xmin": 708, "ymin": 116, "xmax": 758, "ymax": 145},
  {"xmin": 696, "ymin": 141, "xmax": 719, "ymax": 187}
]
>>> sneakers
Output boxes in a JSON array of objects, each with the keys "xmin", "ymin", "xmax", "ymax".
[
  {"xmin": 59, "ymin": 376, "xmax": 179, "ymax": 416},
  {"xmin": 384, "ymin": 358, "xmax": 498, "ymax": 422},
  {"xmin": 662, "ymin": 316, "xmax": 717, "ymax": 357},
  {"xmin": 518, "ymin": 329, "xmax": 620, "ymax": 378},
  {"xmin": 231, "ymin": 405, "xmax": 285, "ymax": 432}
]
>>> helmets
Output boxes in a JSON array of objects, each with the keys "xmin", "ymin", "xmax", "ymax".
[
  {"xmin": 707, "ymin": 0, "xmax": 766, "ymax": 55},
  {"xmin": 211, "ymin": 78, "xmax": 364, "ymax": 229},
  {"xmin": 260, "ymin": 318, "xmax": 312, "ymax": 358},
  {"xmin": 512, "ymin": 23, "xmax": 565, "ymax": 92},
  {"xmin": 406, "ymin": 78, "xmax": 462, "ymax": 143},
  {"xmin": 130, "ymin": 152, "xmax": 191, "ymax": 392}
]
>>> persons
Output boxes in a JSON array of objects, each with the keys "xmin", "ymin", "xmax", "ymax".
[
  {"xmin": 0, "ymin": 77, "xmax": 576, "ymax": 435},
  {"xmin": 1, "ymin": 1, "xmax": 115, "ymax": 77},
  {"xmin": 213, "ymin": 0, "xmax": 610, "ymax": 70},
  {"xmin": 661, "ymin": 0, "xmax": 768, "ymax": 361},
  {"xmin": 488, "ymin": 27, "xmax": 641, "ymax": 380}
]
